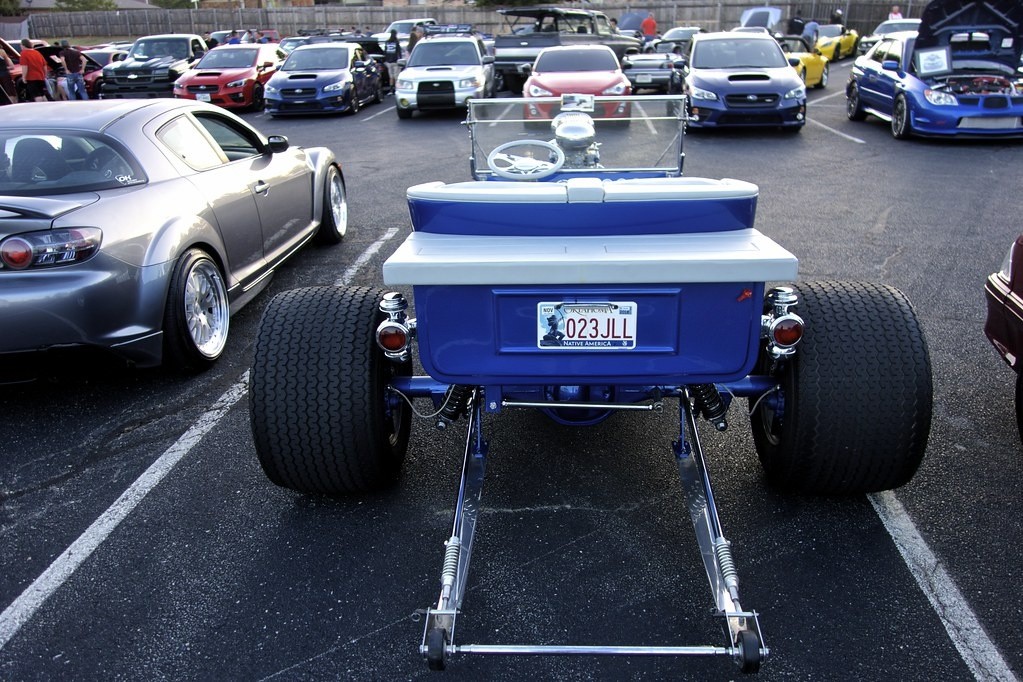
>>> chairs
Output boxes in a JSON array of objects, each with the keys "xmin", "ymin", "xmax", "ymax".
[
  {"xmin": 81, "ymin": 146, "xmax": 132, "ymax": 176},
  {"xmin": 11, "ymin": 138, "xmax": 73, "ymax": 181},
  {"xmin": 735, "ymin": 46, "xmax": 762, "ymax": 62}
]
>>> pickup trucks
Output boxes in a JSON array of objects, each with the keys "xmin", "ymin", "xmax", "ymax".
[{"xmin": 494, "ymin": 8, "xmax": 641, "ymax": 95}]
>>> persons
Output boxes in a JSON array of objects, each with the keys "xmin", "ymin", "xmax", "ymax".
[
  {"xmin": 610, "ymin": 18, "xmax": 620, "ymax": 34},
  {"xmin": 340, "ymin": 26, "xmax": 424, "ymax": 96},
  {"xmin": 787, "ymin": 9, "xmax": 804, "ymax": 36},
  {"xmin": 799, "ymin": 18, "xmax": 819, "ymax": 49},
  {"xmin": 888, "ymin": 6, "xmax": 903, "ymax": 20},
  {"xmin": 19, "ymin": 39, "xmax": 47, "ymax": 102},
  {"xmin": 59, "ymin": 40, "xmax": 89, "ymax": 100},
  {"xmin": 641, "ymin": 12, "xmax": 656, "ymax": 41},
  {"xmin": 203, "ymin": 31, "xmax": 219, "ymax": 50},
  {"xmin": 224, "ymin": 28, "xmax": 267, "ymax": 45},
  {"xmin": 830, "ymin": 9, "xmax": 842, "ymax": 24},
  {"xmin": 0, "ymin": 49, "xmax": 16, "ymax": 104}
]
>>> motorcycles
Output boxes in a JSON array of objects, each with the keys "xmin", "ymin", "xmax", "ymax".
[{"xmin": 246, "ymin": 93, "xmax": 933, "ymax": 493}]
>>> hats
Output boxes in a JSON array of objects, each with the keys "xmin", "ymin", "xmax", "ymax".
[
  {"xmin": 837, "ymin": 10, "xmax": 843, "ymax": 15},
  {"xmin": 62, "ymin": 40, "xmax": 68, "ymax": 47},
  {"xmin": 231, "ymin": 30, "xmax": 238, "ymax": 34}
]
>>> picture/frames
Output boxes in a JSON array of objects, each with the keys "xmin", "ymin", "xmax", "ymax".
[{"xmin": 914, "ymin": 45, "xmax": 953, "ymax": 78}]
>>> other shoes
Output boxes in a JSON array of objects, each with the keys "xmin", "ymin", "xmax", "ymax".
[{"xmin": 387, "ymin": 92, "xmax": 393, "ymax": 96}]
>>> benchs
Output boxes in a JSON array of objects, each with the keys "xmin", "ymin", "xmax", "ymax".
[{"xmin": 405, "ymin": 177, "xmax": 759, "ymax": 236}]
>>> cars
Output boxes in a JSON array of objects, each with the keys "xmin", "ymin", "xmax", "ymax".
[
  {"xmin": 521, "ymin": 45, "xmax": 632, "ymax": 128},
  {"xmin": 772, "ymin": 34, "xmax": 829, "ymax": 89},
  {"xmin": 619, "ymin": 30, "xmax": 647, "ymax": 45},
  {"xmin": 665, "ymin": 29, "xmax": 807, "ymax": 135},
  {"xmin": 394, "ymin": 33, "xmax": 495, "ymax": 120},
  {"xmin": 858, "ymin": 18, "xmax": 922, "ymax": 54},
  {"xmin": 622, "ymin": 39, "xmax": 693, "ymax": 95},
  {"xmin": 642, "ymin": 27, "xmax": 709, "ymax": 53},
  {"xmin": 0, "ymin": 17, "xmax": 505, "ymax": 102},
  {"xmin": 844, "ymin": 0, "xmax": 1023, "ymax": 140},
  {"xmin": 173, "ymin": 43, "xmax": 287, "ymax": 114},
  {"xmin": 263, "ymin": 43, "xmax": 385, "ymax": 118},
  {"xmin": 0, "ymin": 97, "xmax": 348, "ymax": 375},
  {"xmin": 723, "ymin": 7, "xmax": 785, "ymax": 38},
  {"xmin": 813, "ymin": 24, "xmax": 859, "ymax": 64},
  {"xmin": 983, "ymin": 233, "xmax": 1023, "ymax": 440}
]
